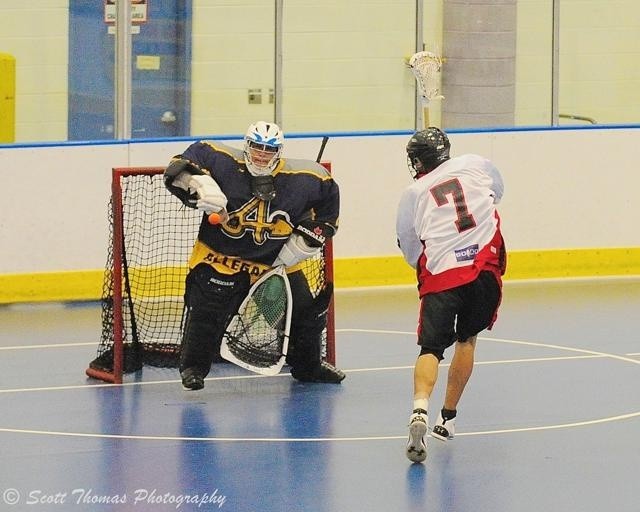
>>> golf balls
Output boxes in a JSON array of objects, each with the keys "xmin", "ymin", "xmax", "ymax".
[{"xmin": 209, "ymin": 213, "xmax": 219, "ymax": 224}]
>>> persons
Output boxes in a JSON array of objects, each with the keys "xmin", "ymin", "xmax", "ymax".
[
  {"xmin": 164, "ymin": 121, "xmax": 346, "ymax": 390},
  {"xmin": 396, "ymin": 127, "xmax": 506, "ymax": 462}
]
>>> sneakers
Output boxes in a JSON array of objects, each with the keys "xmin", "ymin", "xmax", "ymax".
[
  {"xmin": 180, "ymin": 366, "xmax": 204, "ymax": 390},
  {"xmin": 430, "ymin": 406, "xmax": 457, "ymax": 442},
  {"xmin": 290, "ymin": 360, "xmax": 346, "ymax": 383},
  {"xmin": 405, "ymin": 408, "xmax": 429, "ymax": 464}
]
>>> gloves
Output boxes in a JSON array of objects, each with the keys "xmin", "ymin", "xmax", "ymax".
[
  {"xmin": 189, "ymin": 174, "xmax": 230, "ymax": 224},
  {"xmin": 271, "ymin": 218, "xmax": 338, "ymax": 268}
]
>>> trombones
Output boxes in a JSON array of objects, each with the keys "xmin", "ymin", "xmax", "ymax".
[{"xmin": 220, "ymin": 136, "xmax": 329, "ymax": 377}]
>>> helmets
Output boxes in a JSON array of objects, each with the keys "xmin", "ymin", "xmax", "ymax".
[
  {"xmin": 244, "ymin": 121, "xmax": 284, "ymax": 159},
  {"xmin": 406, "ymin": 127, "xmax": 451, "ymax": 174}
]
